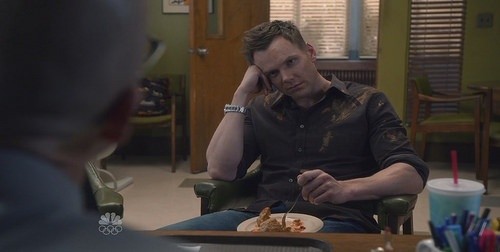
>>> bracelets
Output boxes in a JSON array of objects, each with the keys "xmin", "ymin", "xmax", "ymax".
[{"xmin": 224, "ymin": 102, "xmax": 249, "ymax": 120}]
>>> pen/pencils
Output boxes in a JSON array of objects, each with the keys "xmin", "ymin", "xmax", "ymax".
[{"xmin": 428, "ymin": 208, "xmax": 499, "ymax": 252}]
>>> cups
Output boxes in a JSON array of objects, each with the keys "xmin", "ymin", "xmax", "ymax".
[{"xmin": 426, "ymin": 178, "xmax": 485, "ymax": 232}]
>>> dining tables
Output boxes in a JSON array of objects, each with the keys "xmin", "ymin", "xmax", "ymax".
[{"xmin": 151, "ymin": 230, "xmax": 434, "ymax": 252}]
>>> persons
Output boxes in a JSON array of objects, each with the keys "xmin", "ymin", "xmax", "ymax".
[
  {"xmin": 0, "ymin": 0, "xmax": 195, "ymax": 252},
  {"xmin": 153, "ymin": 19, "xmax": 429, "ymax": 233}
]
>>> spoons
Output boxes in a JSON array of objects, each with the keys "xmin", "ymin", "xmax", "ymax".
[{"xmin": 282, "ymin": 184, "xmax": 303, "ymax": 228}]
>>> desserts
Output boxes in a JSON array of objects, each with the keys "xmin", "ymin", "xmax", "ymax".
[{"xmin": 253, "ymin": 207, "xmax": 291, "ymax": 233}]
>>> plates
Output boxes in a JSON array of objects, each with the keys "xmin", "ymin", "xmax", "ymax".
[{"xmin": 237, "ymin": 213, "xmax": 325, "ymax": 233}]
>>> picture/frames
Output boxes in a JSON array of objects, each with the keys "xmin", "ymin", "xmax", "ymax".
[{"xmin": 162, "ymin": 0, "xmax": 190, "ymax": 13}]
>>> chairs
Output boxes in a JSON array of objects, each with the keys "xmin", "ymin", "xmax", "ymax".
[
  {"xmin": 194, "ymin": 164, "xmax": 418, "ymax": 234},
  {"xmin": 409, "ymin": 84, "xmax": 500, "ymax": 195},
  {"xmin": 99, "ymin": 75, "xmax": 186, "ymax": 174},
  {"xmin": 84, "ymin": 158, "xmax": 124, "ymax": 221}
]
